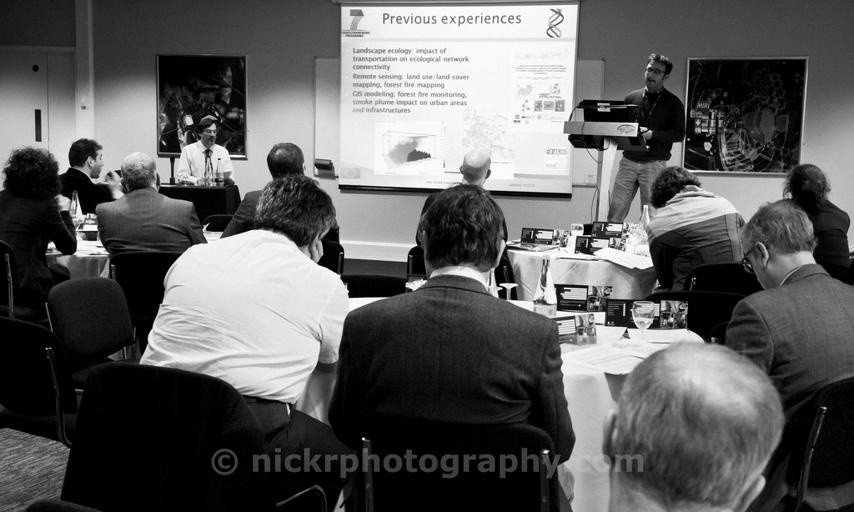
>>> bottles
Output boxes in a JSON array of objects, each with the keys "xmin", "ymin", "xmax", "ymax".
[
  {"xmin": 640, "ymin": 202, "xmax": 649, "ymax": 254},
  {"xmin": 68, "ymin": 189, "xmax": 83, "ymax": 225},
  {"xmin": 205, "ymin": 157, "xmax": 225, "ymax": 187},
  {"xmin": 533, "ymin": 256, "xmax": 558, "ymax": 308}
]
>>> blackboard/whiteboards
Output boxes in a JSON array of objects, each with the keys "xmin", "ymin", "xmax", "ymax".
[{"xmin": 313, "ymin": 55, "xmax": 607, "ymax": 187}]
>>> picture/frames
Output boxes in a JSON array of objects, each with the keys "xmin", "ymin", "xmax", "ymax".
[
  {"xmin": 681, "ymin": 56, "xmax": 810, "ymax": 176},
  {"xmin": 156, "ymin": 54, "xmax": 248, "ymax": 160}
]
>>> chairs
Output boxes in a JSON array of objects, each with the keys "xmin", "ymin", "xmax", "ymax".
[
  {"xmin": 621, "ymin": 291, "xmax": 746, "ymax": 345},
  {"xmin": 684, "ymin": 262, "xmax": 766, "ymax": 298},
  {"xmin": 766, "ymin": 377, "xmax": 854, "ymax": 512},
  {"xmin": 105, "ymin": 169, "xmax": 160, "ymax": 186}
]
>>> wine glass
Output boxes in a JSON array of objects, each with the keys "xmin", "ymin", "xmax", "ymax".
[
  {"xmin": 631, "ymin": 301, "xmax": 656, "ymax": 341},
  {"xmin": 489, "ymin": 281, "xmax": 518, "ymax": 301}
]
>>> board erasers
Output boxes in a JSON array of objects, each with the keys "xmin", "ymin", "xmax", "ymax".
[{"xmin": 314, "ymin": 159, "xmax": 332, "ymax": 170}]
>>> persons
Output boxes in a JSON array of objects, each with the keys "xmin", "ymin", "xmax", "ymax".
[{"xmin": 0, "ymin": 53, "xmax": 854, "ymax": 512}]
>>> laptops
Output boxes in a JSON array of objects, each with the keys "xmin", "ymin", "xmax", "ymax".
[{"xmin": 584, "ymin": 98, "xmax": 636, "ymax": 123}]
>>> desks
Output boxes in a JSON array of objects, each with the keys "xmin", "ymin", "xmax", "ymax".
[
  {"xmin": 294, "ymin": 295, "xmax": 705, "ymax": 512},
  {"xmin": 507, "ymin": 231, "xmax": 661, "ymax": 303},
  {"xmin": 159, "ymin": 181, "xmax": 240, "ymax": 231}
]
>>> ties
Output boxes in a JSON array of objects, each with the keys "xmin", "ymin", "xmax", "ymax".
[{"xmin": 205, "ymin": 150, "xmax": 213, "ymax": 176}]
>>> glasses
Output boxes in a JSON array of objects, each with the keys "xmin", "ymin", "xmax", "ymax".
[{"xmin": 739, "ymin": 245, "xmax": 768, "ymax": 275}]
